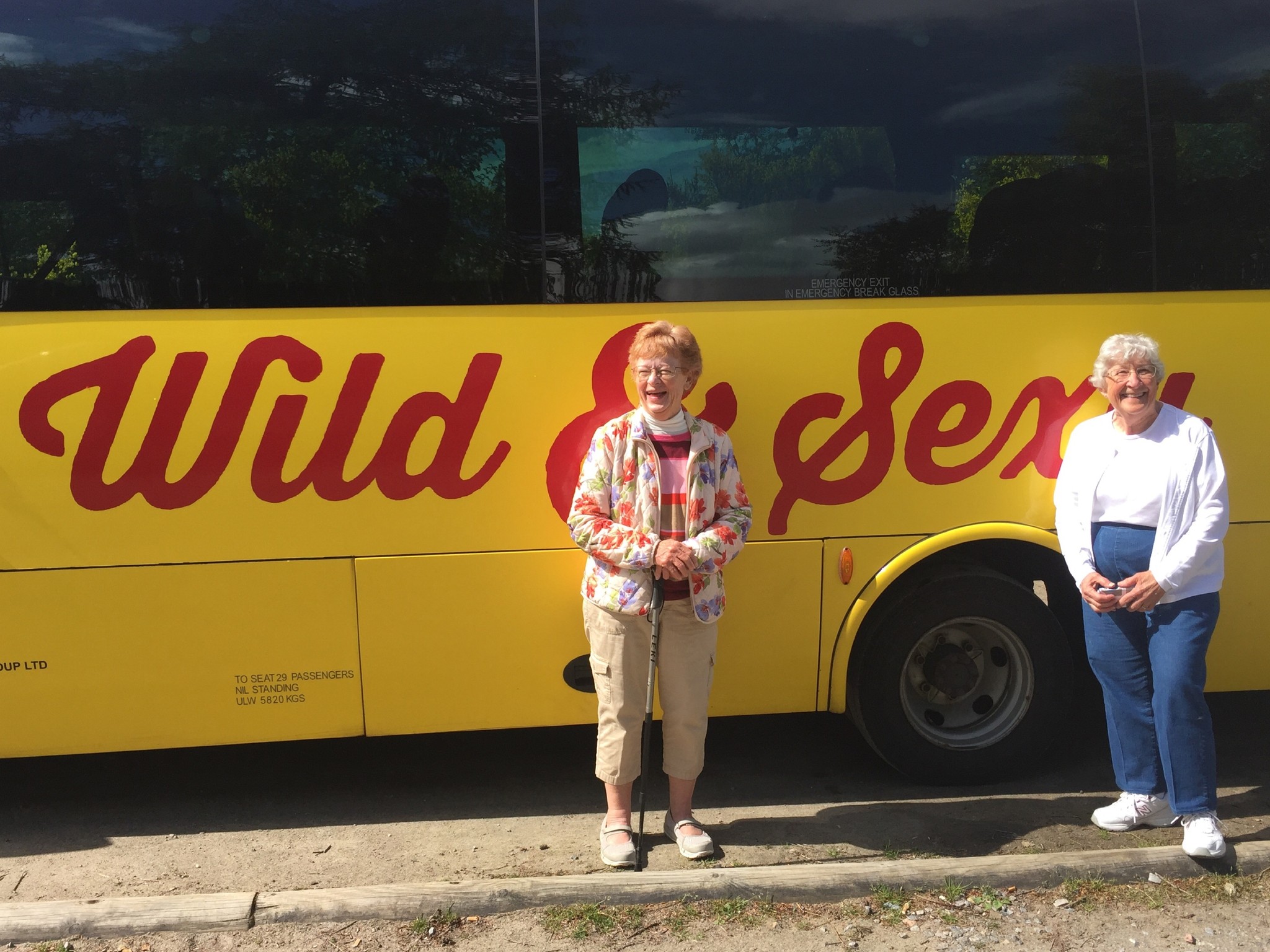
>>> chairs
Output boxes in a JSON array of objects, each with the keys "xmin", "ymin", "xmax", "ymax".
[{"xmin": 601, "ymin": 168, "xmax": 668, "ymax": 239}]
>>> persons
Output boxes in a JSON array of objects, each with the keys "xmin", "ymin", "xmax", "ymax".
[
  {"xmin": 566, "ymin": 320, "xmax": 753, "ymax": 868},
  {"xmin": 1053, "ymin": 333, "xmax": 1225, "ymax": 857}
]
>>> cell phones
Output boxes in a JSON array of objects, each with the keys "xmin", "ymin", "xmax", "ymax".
[{"xmin": 1098, "ymin": 586, "xmax": 1139, "ymax": 608}]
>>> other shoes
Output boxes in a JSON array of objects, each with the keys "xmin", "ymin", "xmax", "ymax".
[
  {"xmin": 599, "ymin": 813, "xmax": 636, "ymax": 867},
  {"xmin": 663, "ymin": 808, "xmax": 714, "ymax": 858}
]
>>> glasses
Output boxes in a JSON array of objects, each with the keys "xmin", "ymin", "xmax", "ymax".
[
  {"xmin": 1102, "ymin": 365, "xmax": 1159, "ymax": 379},
  {"xmin": 631, "ymin": 364, "xmax": 689, "ymax": 379}
]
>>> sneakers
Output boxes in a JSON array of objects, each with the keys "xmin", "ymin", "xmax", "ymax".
[
  {"xmin": 1091, "ymin": 792, "xmax": 1178, "ymax": 832},
  {"xmin": 1181, "ymin": 810, "xmax": 1228, "ymax": 858}
]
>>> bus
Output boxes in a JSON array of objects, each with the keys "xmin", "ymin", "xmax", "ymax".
[{"xmin": 0, "ymin": 1, "xmax": 1269, "ymax": 788}]
